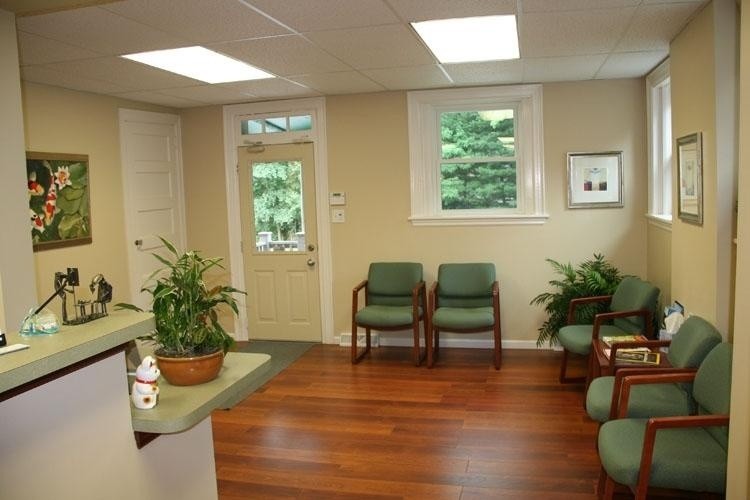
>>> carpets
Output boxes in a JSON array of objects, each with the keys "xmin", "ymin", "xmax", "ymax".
[{"xmin": 207, "ymin": 339, "xmax": 318, "ymax": 411}]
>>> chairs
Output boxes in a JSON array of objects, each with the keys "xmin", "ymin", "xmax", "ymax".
[
  {"xmin": 557, "ymin": 276, "xmax": 660, "ymax": 380},
  {"xmin": 583, "ymin": 315, "xmax": 721, "ymax": 500},
  {"xmin": 597, "ymin": 334, "xmax": 735, "ymax": 499},
  {"xmin": 426, "ymin": 263, "xmax": 502, "ymax": 367},
  {"xmin": 351, "ymin": 262, "xmax": 427, "ymax": 366}
]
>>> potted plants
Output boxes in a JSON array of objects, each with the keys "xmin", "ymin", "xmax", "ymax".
[{"xmin": 114, "ymin": 237, "xmax": 249, "ymax": 385}]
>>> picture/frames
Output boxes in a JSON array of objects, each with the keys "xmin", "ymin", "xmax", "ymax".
[
  {"xmin": 27, "ymin": 154, "xmax": 94, "ymax": 252},
  {"xmin": 566, "ymin": 152, "xmax": 622, "ymax": 208},
  {"xmin": 677, "ymin": 133, "xmax": 703, "ymax": 228}
]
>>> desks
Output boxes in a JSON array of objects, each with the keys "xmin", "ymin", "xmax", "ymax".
[{"xmin": 590, "ymin": 334, "xmax": 672, "ymax": 378}]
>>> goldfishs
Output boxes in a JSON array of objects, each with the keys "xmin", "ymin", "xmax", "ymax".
[
  {"xmin": 43, "ymin": 161, "xmax": 61, "ymax": 225},
  {"xmin": 28, "ymin": 172, "xmax": 44, "ymax": 202},
  {"xmin": 30, "ymin": 207, "xmax": 46, "ymax": 235}
]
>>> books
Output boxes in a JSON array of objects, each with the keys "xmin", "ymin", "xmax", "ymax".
[{"xmin": 602, "ymin": 332, "xmax": 661, "ymax": 365}]
